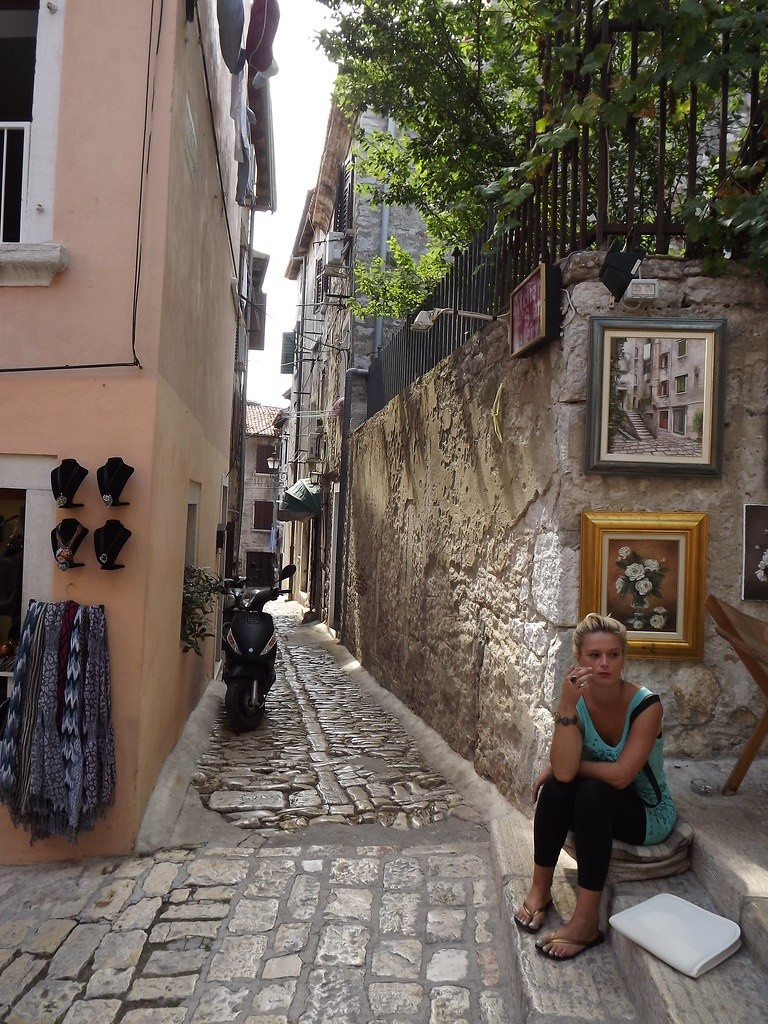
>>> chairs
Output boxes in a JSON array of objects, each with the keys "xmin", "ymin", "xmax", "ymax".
[{"xmin": 705, "ymin": 592, "xmax": 768, "ymax": 795}]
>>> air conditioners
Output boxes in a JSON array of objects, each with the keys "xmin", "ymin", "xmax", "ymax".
[
  {"xmin": 307, "ymin": 433, "xmax": 325, "ymax": 462},
  {"xmin": 323, "ymin": 232, "xmax": 344, "ymax": 276}
]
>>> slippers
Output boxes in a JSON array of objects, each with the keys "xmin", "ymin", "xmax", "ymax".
[
  {"xmin": 514, "ymin": 897, "xmax": 553, "ymax": 934},
  {"xmin": 535, "ymin": 929, "xmax": 604, "ymax": 961}
]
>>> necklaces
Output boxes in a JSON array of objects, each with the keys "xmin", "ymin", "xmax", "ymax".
[
  {"xmin": 56, "ymin": 466, "xmax": 81, "ymax": 506},
  {"xmin": 56, "ymin": 522, "xmax": 84, "ymax": 571},
  {"xmin": 99, "ymin": 524, "xmax": 124, "ymax": 564},
  {"xmin": 102, "ymin": 462, "xmax": 124, "ymax": 508}
]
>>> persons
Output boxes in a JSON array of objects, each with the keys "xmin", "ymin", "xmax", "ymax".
[
  {"xmin": 91, "ymin": 518, "xmax": 134, "ymax": 571},
  {"xmin": 514, "ymin": 612, "xmax": 677, "ymax": 961},
  {"xmin": 50, "ymin": 517, "xmax": 89, "ymax": 570},
  {"xmin": 50, "ymin": 457, "xmax": 91, "ymax": 509},
  {"xmin": 96, "ymin": 456, "xmax": 136, "ymax": 507}
]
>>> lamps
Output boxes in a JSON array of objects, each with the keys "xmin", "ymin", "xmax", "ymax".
[
  {"xmin": 411, "ymin": 307, "xmax": 509, "ymax": 331},
  {"xmin": 267, "ymin": 456, "xmax": 287, "ymax": 487},
  {"xmin": 310, "ymin": 469, "xmax": 322, "ymax": 485},
  {"xmin": 597, "ymin": 239, "xmax": 648, "ymax": 303}
]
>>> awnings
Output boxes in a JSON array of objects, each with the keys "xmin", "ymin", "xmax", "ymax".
[{"xmin": 276, "ymin": 477, "xmax": 321, "ymax": 513}]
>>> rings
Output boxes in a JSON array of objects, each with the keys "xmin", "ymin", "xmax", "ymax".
[{"xmin": 569, "ymin": 676, "xmax": 578, "ymax": 684}]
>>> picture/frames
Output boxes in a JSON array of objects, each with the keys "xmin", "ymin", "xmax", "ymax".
[
  {"xmin": 579, "ymin": 511, "xmax": 708, "ymax": 661},
  {"xmin": 585, "ymin": 315, "xmax": 727, "ymax": 479}
]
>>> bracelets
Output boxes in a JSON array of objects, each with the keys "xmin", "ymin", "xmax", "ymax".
[{"xmin": 554, "ymin": 711, "xmax": 578, "ymax": 727}]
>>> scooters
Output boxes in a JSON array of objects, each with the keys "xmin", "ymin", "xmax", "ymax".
[{"xmin": 215, "ymin": 564, "xmax": 297, "ymax": 731}]
